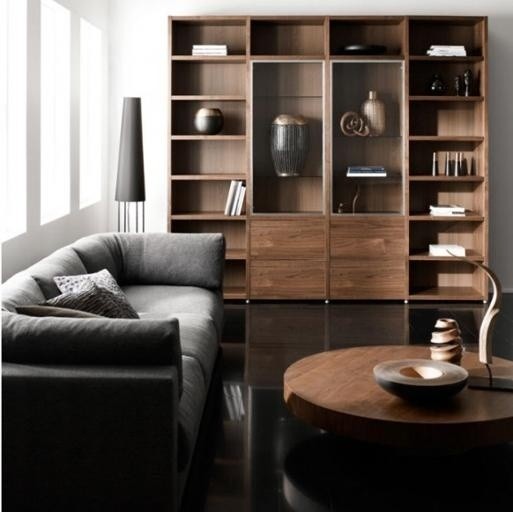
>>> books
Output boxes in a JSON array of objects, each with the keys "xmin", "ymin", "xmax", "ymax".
[
  {"xmin": 192, "ymin": 44, "xmax": 228, "ymax": 56},
  {"xmin": 347, "ymin": 166, "xmax": 387, "ymax": 178},
  {"xmin": 429, "ymin": 204, "xmax": 466, "ymax": 217},
  {"xmin": 224, "ymin": 181, "xmax": 246, "ymax": 216},
  {"xmin": 427, "ymin": 45, "xmax": 466, "ymax": 57}
]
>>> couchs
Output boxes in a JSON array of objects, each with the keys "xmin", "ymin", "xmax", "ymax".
[{"xmin": 1, "ymin": 232, "xmax": 226, "ymax": 507}]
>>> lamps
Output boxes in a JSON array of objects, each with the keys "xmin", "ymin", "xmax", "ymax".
[{"xmin": 115, "ymin": 98, "xmax": 146, "ymax": 232}]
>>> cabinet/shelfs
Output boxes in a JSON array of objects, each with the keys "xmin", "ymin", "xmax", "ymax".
[
  {"xmin": 248, "ymin": 15, "xmax": 327, "ymax": 304},
  {"xmin": 407, "ymin": 14, "xmax": 488, "ymax": 307},
  {"xmin": 328, "ymin": 14, "xmax": 409, "ymax": 306},
  {"xmin": 167, "ymin": 15, "xmax": 250, "ymax": 304}
]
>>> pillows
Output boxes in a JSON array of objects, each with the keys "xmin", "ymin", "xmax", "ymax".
[
  {"xmin": 14, "ymin": 304, "xmax": 104, "ymax": 319},
  {"xmin": 39, "ymin": 284, "xmax": 140, "ymax": 319},
  {"xmin": 53, "ymin": 268, "xmax": 130, "ymax": 311}
]
